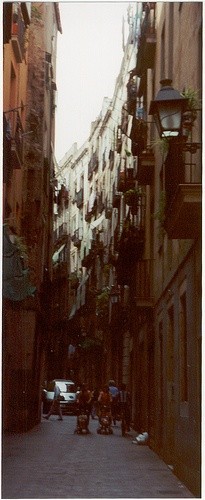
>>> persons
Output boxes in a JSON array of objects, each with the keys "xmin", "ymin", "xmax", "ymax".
[
  {"xmin": 43, "ymin": 379, "xmax": 65, "ymax": 421},
  {"xmin": 72, "ymin": 382, "xmax": 133, "ymax": 437}
]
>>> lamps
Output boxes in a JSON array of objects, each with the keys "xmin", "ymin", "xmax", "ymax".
[{"xmin": 148, "ymin": 77, "xmax": 190, "ymax": 138}]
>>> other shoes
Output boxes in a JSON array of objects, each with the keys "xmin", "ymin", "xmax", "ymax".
[
  {"xmin": 43, "ymin": 416, "xmax": 48, "ymax": 419},
  {"xmin": 58, "ymin": 418, "xmax": 63, "ymax": 421}
]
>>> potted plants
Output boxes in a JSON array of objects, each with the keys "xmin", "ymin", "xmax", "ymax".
[
  {"xmin": 123, "ymin": 188, "xmax": 142, "ymax": 206},
  {"xmin": 182, "ymin": 84, "xmax": 202, "ymax": 138}
]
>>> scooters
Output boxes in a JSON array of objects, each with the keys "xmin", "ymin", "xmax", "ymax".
[
  {"xmin": 97, "ymin": 405, "xmax": 114, "ymax": 434},
  {"xmin": 73, "ymin": 404, "xmax": 90, "ymax": 434}
]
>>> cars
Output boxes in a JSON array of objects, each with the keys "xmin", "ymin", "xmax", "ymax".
[{"xmin": 42, "ymin": 379, "xmax": 80, "ymax": 414}]
